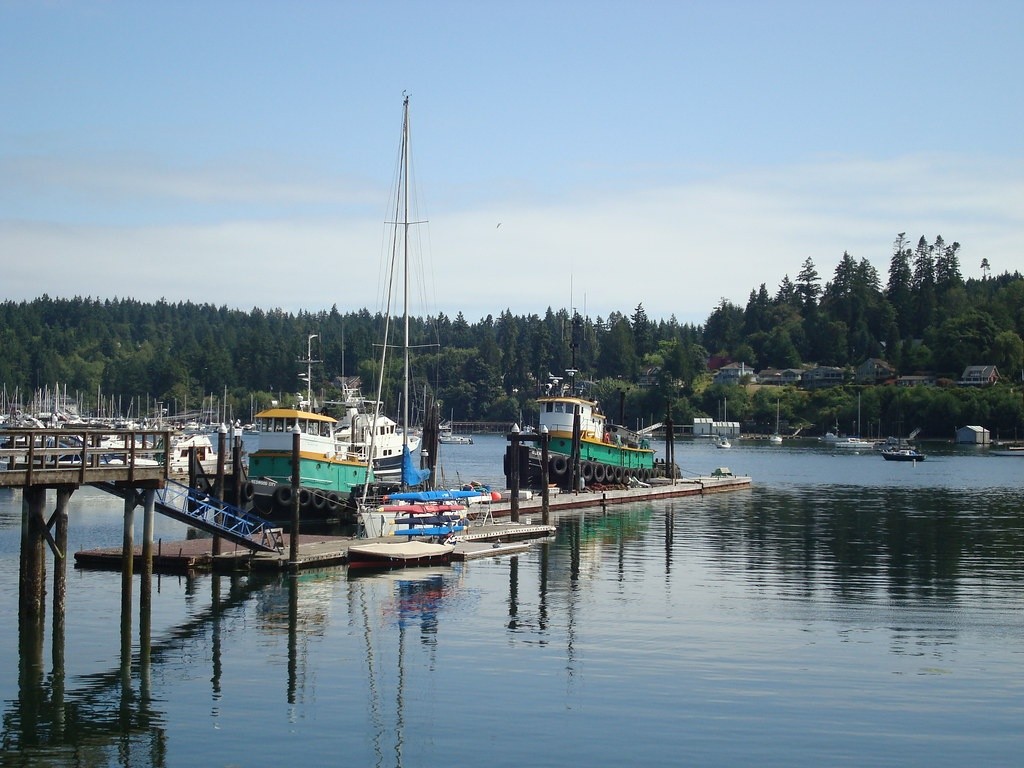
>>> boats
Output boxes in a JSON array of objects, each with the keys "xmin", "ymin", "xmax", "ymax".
[
  {"xmin": 880, "ymin": 449, "xmax": 928, "ymax": 462},
  {"xmin": 769, "ymin": 434, "xmax": 783, "ymax": 442},
  {"xmin": 835, "ymin": 437, "xmax": 876, "ymax": 448},
  {"xmin": 503, "ymin": 272, "xmax": 659, "ymax": 492}
]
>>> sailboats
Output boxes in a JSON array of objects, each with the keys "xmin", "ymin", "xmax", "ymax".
[
  {"xmin": 0, "ymin": 86, "xmax": 476, "ymax": 530},
  {"xmin": 713, "ymin": 396, "xmax": 732, "ymax": 448}
]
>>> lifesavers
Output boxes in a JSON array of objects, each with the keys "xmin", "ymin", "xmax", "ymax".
[
  {"xmin": 299, "ymin": 487, "xmax": 311, "ymax": 509},
  {"xmin": 326, "ymin": 493, "xmax": 339, "ymax": 512},
  {"xmin": 312, "ymin": 489, "xmax": 326, "ymax": 512},
  {"xmin": 242, "ymin": 481, "xmax": 254, "ymax": 502},
  {"xmin": 581, "ymin": 460, "xmax": 594, "ymax": 483},
  {"xmin": 623, "ymin": 468, "xmax": 665, "ymax": 485},
  {"xmin": 550, "ymin": 455, "xmax": 568, "ymax": 476},
  {"xmin": 277, "ymin": 485, "xmax": 292, "ymax": 507},
  {"xmin": 604, "ymin": 433, "xmax": 610, "ymax": 443},
  {"xmin": 614, "ymin": 466, "xmax": 623, "ymax": 484},
  {"xmin": 604, "ymin": 464, "xmax": 615, "ymax": 483},
  {"xmin": 594, "ymin": 463, "xmax": 606, "ymax": 483}
]
